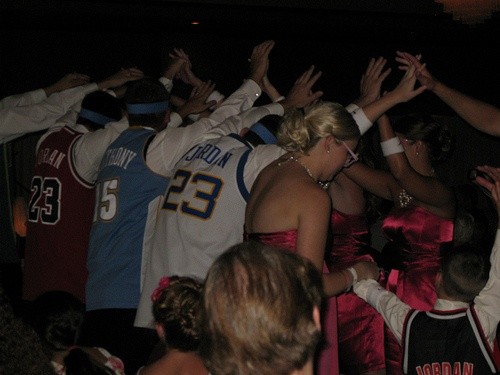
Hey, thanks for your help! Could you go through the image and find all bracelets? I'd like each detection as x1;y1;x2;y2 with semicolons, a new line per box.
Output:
342;271;350;291
348;267;357;284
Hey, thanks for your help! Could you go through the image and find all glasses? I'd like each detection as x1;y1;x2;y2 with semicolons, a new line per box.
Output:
336;138;359;167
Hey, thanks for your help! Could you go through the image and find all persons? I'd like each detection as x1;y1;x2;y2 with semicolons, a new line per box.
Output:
200;243;322;375
137;275;213;374
23;57;186;304
353;165;500;375
82;41;273;375
238;44;383;375
73;49;225;124
0;67;144;145
301;88;454;375
22;289;125;375
395;0;500;137
1;316;45;375
130;54;427;375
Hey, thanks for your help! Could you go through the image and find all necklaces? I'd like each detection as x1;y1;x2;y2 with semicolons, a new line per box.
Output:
276;155;330;191
397;166;434;207
